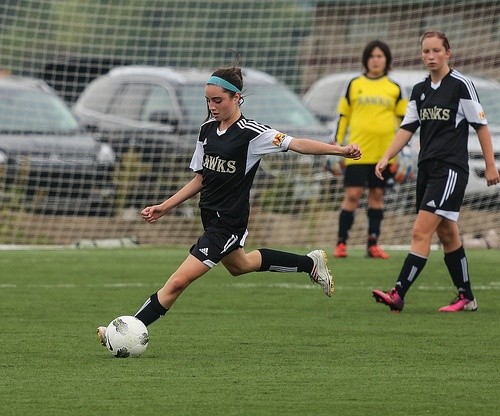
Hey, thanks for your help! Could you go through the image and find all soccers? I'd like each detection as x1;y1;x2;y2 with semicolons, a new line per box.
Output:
105;316;149;356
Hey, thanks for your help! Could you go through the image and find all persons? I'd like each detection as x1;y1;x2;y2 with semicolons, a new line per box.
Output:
96;68;362;346
372;32;500;313
325;40;409;260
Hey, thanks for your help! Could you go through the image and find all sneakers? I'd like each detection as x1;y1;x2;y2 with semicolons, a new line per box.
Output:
372;288;404;313
96;326;106;346
306;250;335;298
334;243;348;257
367;245;389;258
440;292;478;313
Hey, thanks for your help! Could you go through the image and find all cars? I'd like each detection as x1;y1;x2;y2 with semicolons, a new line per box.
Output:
70;63;334;214
300;69;500;216
0;76;122;217
